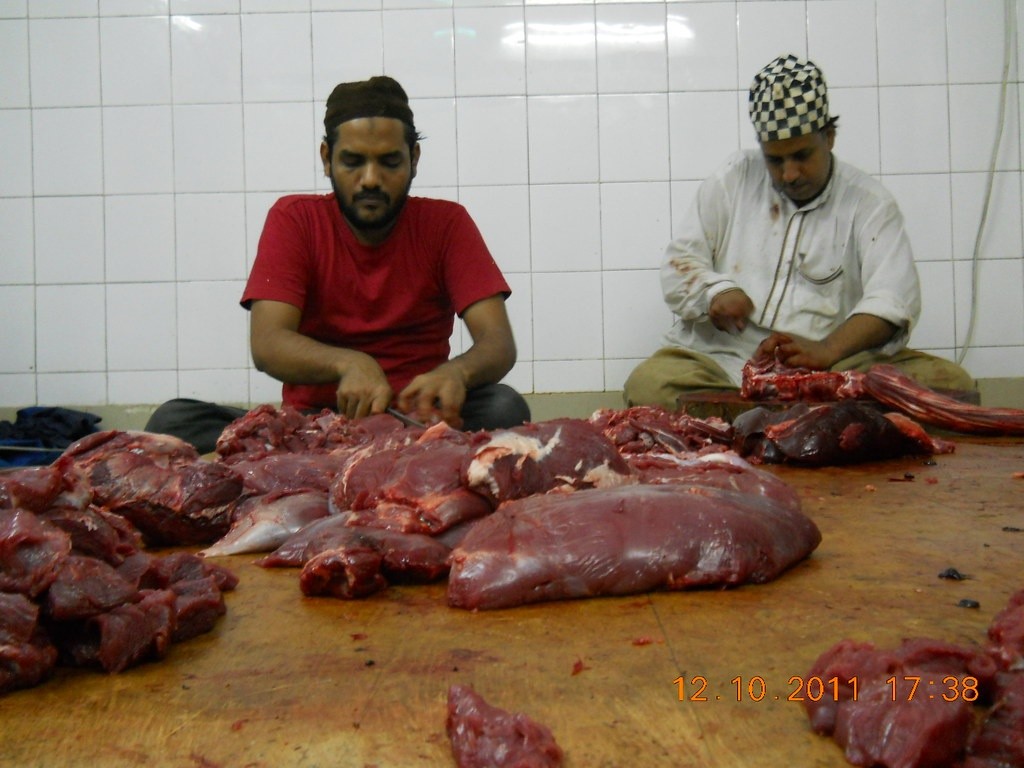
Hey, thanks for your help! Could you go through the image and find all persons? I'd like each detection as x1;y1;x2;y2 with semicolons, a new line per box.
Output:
142;76;532;456
623;54;982;419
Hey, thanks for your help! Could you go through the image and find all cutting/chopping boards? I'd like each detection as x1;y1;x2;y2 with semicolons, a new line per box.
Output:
678;388;984;426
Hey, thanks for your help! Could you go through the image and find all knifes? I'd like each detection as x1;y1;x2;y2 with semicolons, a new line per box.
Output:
386;406;426;429
744;316;795;346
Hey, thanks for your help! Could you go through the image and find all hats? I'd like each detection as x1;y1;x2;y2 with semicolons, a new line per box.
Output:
324;76;414;135
749;54;829;141
